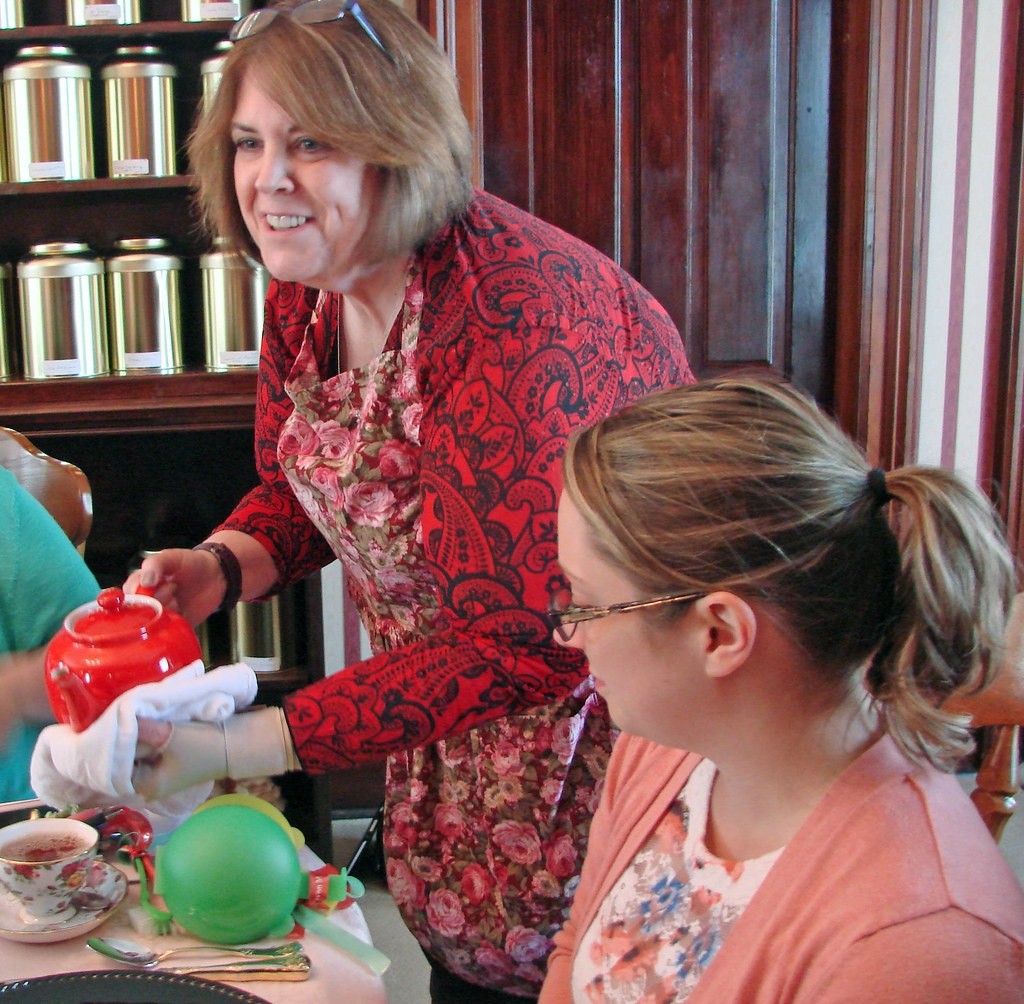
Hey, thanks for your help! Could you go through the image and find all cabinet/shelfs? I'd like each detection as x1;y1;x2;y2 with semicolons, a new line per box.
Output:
0;0;333;866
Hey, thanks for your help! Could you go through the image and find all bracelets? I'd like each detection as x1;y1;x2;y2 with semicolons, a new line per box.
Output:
194;539;244;611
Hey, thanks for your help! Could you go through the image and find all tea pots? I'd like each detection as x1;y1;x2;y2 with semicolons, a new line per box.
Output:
43;576;204;734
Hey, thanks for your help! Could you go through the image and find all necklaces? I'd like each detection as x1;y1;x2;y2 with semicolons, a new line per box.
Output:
329;289;351;375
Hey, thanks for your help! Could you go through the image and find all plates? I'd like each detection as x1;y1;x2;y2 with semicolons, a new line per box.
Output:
0;860;130;944
0;967;274;1004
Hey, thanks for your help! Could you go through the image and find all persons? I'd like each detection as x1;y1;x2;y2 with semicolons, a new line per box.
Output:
0;459;102;819
537;372;1024;1003
130;0;704;1004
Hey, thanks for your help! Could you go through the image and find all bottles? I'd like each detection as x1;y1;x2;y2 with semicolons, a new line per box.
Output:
0;0;270;382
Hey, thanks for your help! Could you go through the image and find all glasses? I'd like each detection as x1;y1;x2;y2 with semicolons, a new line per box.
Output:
546;586;774;642
229;0;393;62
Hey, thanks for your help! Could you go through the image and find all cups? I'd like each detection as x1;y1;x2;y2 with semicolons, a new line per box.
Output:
231;595;282;674
0;818;100;926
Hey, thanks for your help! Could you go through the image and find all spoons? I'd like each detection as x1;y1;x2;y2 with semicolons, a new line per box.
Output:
85;935;304;969
70;890;111;910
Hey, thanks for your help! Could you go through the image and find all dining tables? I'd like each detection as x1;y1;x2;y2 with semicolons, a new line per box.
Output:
0;843;390;1004
